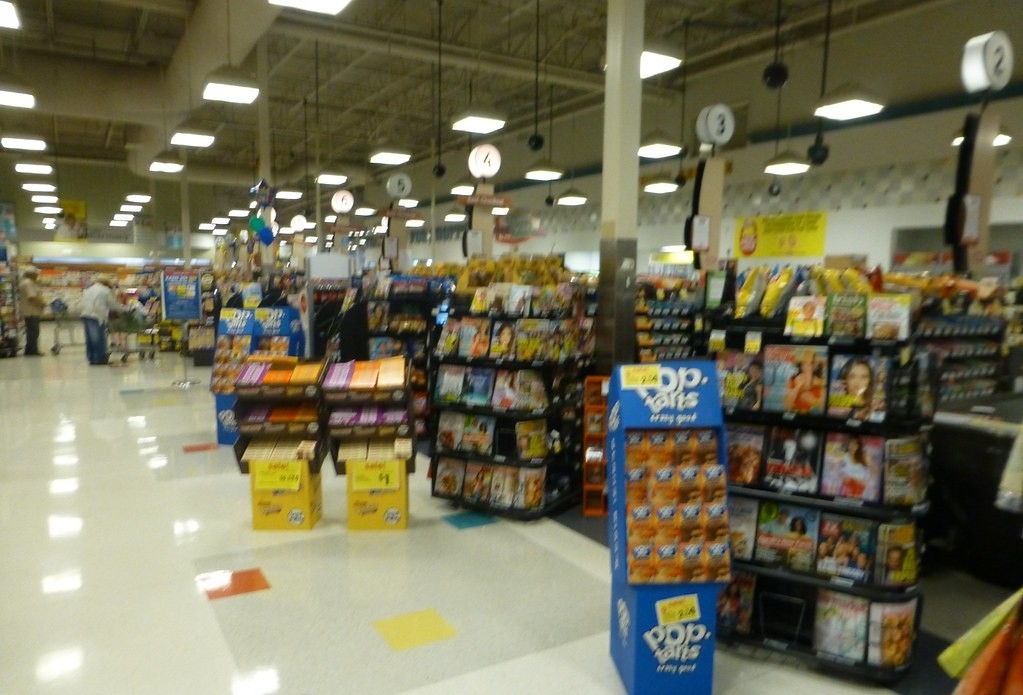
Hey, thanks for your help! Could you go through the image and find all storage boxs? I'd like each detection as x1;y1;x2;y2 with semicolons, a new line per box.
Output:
627;428;733;587
582;375;610;518
214;335;411;533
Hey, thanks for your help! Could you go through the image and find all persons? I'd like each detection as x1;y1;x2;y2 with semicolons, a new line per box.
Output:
503;372;516;400
369;302;387;329
738;360;763;412
492;322;514;357
470;319;490;355
759;509;790;536
784;360;824;410
833;357;874;414
551;332;560;360
474;470;484;490
81;276;121;364
885;547;905;573
786;516;810;563
832;437;875;498
19;265;47;355
789;301;823;337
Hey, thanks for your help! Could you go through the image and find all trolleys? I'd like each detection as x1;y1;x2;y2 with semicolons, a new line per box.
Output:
43;301;88;356
102;310;163;364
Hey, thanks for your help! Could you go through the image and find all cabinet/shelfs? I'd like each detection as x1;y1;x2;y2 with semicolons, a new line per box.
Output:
607;360;734;695
319;355;417;477
253;306;307;359
232;356;328;475
0;261;25;358
213;306;255;446
428;294;576;521
361;291;437;442
706;314;934;688
635;312;695;364
912;317;1007;436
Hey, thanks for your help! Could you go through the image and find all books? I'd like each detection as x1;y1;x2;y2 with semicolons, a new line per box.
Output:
432;283;594;509
366;301;392;360
784;291;909;340
720;346;926;665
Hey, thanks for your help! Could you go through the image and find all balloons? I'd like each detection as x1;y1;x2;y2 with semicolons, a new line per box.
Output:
249;215;265;232
258;228;273;245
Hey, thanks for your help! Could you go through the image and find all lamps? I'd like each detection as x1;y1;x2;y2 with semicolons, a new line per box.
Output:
124;129;152;203
0;81;40;109
812;0;886;121
147;36;186;174
523;84;565;182
449;104;508;136
597;36;685;79
443;198;468;223
169;15;216;148
200;0;260;105
555;166;590;208
367;146;413;166
193;169;427;253
643;159;679;195
0;0;25;31
0;129;49;153
636;74;685;159
448;175;475;197
761;0;810;177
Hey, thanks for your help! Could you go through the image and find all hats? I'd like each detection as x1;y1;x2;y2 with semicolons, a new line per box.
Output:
96;273;110;285
25;265;42;274
